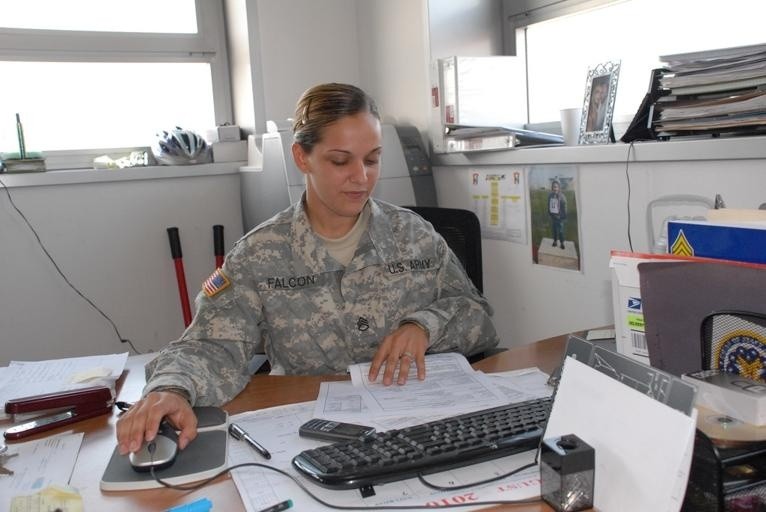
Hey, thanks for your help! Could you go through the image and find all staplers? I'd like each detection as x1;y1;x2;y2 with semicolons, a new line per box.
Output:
3;386;113;440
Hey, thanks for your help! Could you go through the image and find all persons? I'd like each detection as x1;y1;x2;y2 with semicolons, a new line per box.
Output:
547;177;569;251
596;77;609;132
114;80;501;457
585;81;604;132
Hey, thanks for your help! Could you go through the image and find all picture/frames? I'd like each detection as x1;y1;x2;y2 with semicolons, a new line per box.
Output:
577;58;621;144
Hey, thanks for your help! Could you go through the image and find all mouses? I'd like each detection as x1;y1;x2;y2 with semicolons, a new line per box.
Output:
126;422;179;471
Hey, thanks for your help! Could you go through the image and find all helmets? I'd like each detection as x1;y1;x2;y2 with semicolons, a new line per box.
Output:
151;127;211;165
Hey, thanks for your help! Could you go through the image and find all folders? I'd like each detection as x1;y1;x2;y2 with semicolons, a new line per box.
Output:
442;57;566;154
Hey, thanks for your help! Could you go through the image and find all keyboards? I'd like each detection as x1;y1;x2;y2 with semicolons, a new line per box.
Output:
291;395;554;498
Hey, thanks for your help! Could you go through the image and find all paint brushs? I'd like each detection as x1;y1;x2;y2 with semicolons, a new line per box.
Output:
253;499;294;510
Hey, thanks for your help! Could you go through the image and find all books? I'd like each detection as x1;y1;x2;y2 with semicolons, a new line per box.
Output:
650;42;766;140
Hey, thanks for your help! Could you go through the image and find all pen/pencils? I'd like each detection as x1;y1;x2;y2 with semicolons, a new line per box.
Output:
115;402;169;431
229;422;269;460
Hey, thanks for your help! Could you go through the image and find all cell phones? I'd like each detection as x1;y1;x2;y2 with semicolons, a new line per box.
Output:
298;417;377;443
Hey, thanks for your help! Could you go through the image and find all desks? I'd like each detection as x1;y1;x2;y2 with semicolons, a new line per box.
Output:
0;324;766;512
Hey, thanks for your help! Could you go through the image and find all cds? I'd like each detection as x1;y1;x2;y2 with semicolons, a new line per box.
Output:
704;412;746;431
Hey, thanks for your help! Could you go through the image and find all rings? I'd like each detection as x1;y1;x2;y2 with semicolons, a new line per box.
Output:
400;350;415;359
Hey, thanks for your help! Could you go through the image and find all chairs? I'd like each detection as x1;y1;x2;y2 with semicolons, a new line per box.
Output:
401;206;487;363
